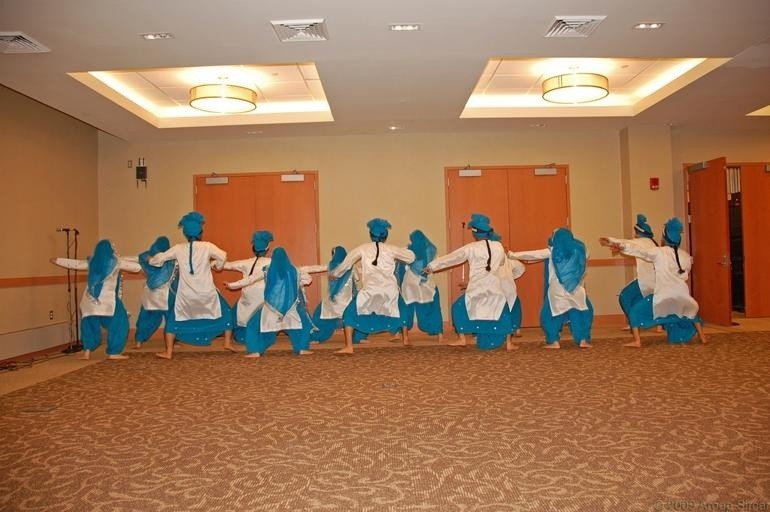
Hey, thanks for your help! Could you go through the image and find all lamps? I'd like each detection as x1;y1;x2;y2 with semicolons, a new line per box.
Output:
188;82;260;113
540;72;611;105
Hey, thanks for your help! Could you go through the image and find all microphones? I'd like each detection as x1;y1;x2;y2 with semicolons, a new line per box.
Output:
73;228;79;234
56;228;71;232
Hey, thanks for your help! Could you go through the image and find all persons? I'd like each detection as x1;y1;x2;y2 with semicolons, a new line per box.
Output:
118;235;175;349
388;230;444;344
210;231;273;345
610;218;706;347
298;246;372;344
49;238;142;360
145;212;240;360
599;213;665;333
331;217;415;356
420;214;594;351
222;247;315;358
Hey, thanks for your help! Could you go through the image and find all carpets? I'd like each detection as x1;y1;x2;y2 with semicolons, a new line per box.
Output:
0;327;770;512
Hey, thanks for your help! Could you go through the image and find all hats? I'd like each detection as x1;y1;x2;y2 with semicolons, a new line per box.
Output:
662;224;682;246
368;225;389;242
633;220;655;238
182;218;203;242
473;221;495;238
253;238;270;256
